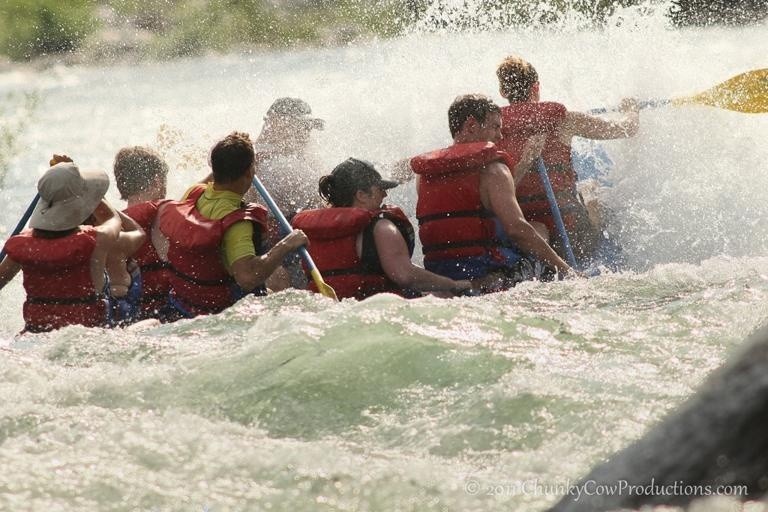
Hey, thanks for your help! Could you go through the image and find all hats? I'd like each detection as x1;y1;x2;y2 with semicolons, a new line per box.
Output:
331;157;398;197
29;162;109;231
262;97;327;131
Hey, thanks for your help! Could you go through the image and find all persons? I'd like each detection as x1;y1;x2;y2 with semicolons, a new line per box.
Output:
82;196;146;328
161;130;310;323
495;56;640;273
292;156;473;303
0;153;121;333
113;146;175;325
242;97;333;293
411;93;583;297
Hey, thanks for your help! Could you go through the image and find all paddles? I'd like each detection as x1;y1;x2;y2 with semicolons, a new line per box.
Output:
253;172;341;302
583;68;767;112
